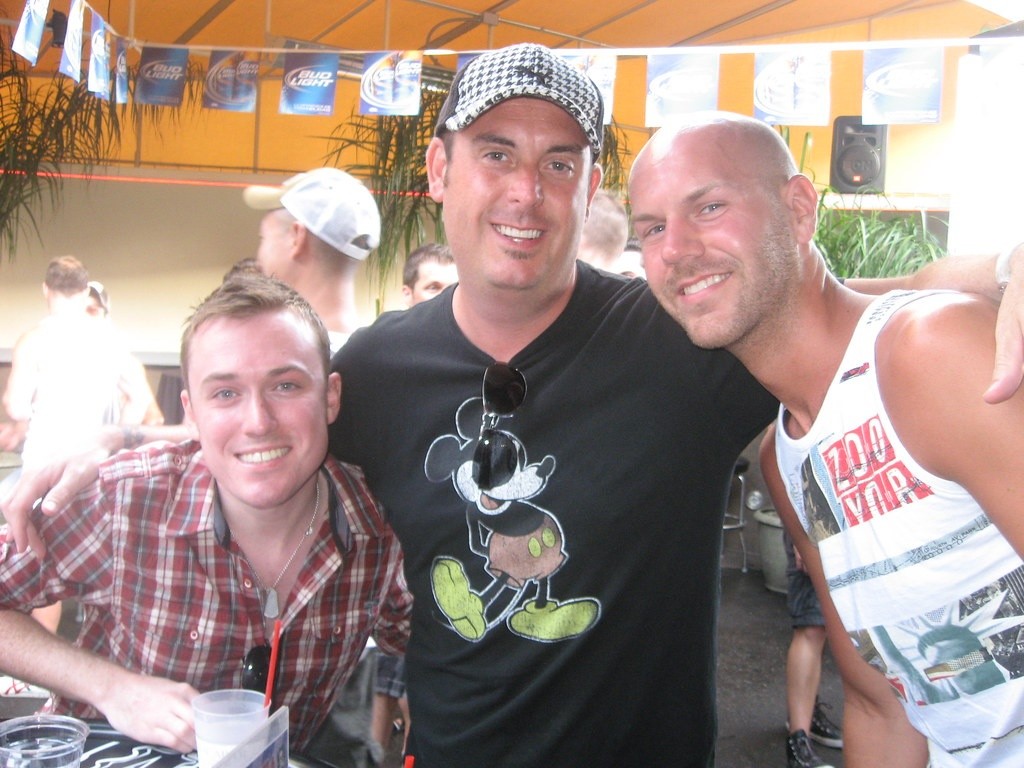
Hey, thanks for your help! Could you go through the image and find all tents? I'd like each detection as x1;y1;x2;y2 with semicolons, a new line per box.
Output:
0;0;1012;202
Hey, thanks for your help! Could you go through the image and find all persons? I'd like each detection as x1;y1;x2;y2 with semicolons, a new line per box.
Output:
784;528;844;768
0;40;1024;768
243;166;381;360
3;255;165;477
627;110;1024;768
401;242;459;307
0;276;413;754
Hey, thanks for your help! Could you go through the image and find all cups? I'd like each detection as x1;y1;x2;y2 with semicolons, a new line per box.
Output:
191;689;271;768
0;715;89;768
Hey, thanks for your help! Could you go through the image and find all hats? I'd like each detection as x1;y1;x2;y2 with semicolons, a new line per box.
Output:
433;41;606;171
88;280;110;314
242;166;383;260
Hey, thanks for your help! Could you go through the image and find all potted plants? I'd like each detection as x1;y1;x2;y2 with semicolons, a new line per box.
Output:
752;122;952;595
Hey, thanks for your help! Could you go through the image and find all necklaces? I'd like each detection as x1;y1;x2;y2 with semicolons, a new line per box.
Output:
217;478;319;619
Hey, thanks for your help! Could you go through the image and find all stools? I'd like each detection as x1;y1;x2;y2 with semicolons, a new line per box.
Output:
723;454;752;573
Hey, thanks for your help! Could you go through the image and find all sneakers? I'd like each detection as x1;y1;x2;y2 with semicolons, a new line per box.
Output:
810;695;844;748
785;728;835;768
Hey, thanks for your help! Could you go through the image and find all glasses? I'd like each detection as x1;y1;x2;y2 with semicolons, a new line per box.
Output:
471;361;529;491
241;640;281;714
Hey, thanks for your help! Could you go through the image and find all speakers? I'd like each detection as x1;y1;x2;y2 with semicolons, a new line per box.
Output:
830;116;887;195
